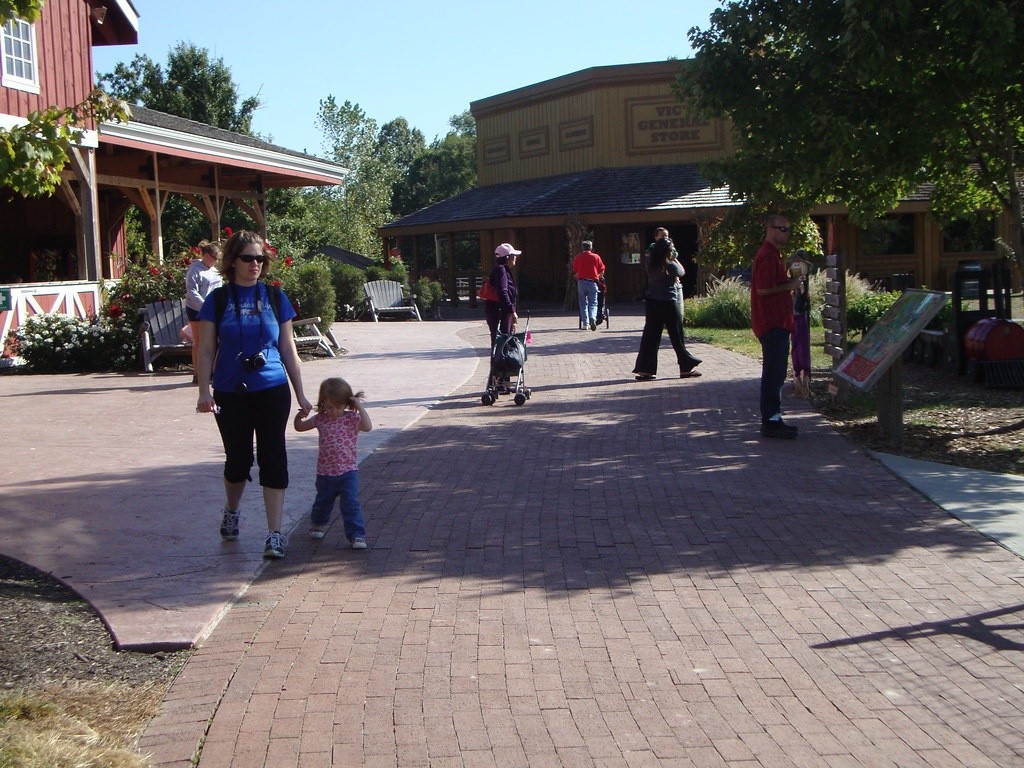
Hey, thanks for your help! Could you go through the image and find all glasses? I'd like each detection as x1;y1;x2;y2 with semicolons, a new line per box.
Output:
236;253;266;263
771;224;792;233
208;253;221;263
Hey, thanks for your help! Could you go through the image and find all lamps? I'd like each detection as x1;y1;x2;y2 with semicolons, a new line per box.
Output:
90;5;107;24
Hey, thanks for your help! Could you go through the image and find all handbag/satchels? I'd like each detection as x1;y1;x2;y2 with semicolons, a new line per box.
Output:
493;335;526;372
479;282;501;302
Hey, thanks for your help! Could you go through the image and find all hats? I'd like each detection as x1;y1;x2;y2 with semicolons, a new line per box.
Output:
495;243;522;258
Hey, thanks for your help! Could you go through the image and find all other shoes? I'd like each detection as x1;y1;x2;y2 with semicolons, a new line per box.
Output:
690;355;703;367
192;376;198;384
582;325;588;329
209;374;214;383
589;318;596;331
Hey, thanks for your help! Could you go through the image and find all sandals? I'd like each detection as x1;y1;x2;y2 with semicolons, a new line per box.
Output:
635;371;656;380
680;371;702;378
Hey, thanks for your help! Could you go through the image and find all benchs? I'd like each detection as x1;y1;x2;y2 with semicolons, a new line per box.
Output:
137;299;193;372
359;280;423;323
288;316;336;358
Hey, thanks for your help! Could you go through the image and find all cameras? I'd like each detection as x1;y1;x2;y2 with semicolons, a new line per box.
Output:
243;352;267;372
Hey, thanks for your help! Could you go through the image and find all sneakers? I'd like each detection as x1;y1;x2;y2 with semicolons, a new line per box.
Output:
760;419;798;438
264;530;288;559
219;504;245;540
352;536;367;549
308;520;324;538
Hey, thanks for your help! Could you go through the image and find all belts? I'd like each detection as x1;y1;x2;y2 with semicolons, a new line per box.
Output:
578;278;594;282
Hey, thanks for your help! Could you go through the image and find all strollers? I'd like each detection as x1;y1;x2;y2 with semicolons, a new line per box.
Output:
481;309;532;406
577;276;610;330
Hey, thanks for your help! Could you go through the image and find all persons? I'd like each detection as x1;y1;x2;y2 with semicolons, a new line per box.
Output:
185;244;222;385
786;249;818;399
632;227;703;381
484;243;521;395
196;230;312;558
295;378;372;548
572;240;605;331
751;215;802;438
